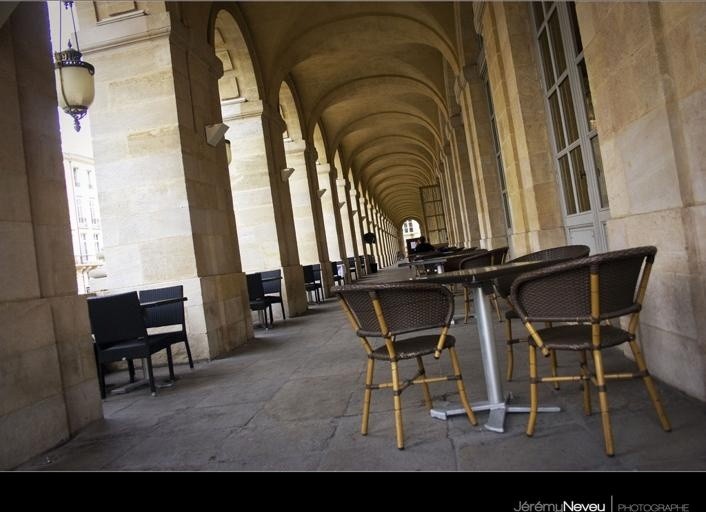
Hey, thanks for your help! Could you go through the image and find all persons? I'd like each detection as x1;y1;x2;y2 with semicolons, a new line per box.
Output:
416;236;433;252
414;238;421;252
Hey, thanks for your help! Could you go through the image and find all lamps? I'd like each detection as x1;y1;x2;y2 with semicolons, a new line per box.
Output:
224;138;231;166
52;1;95;133
